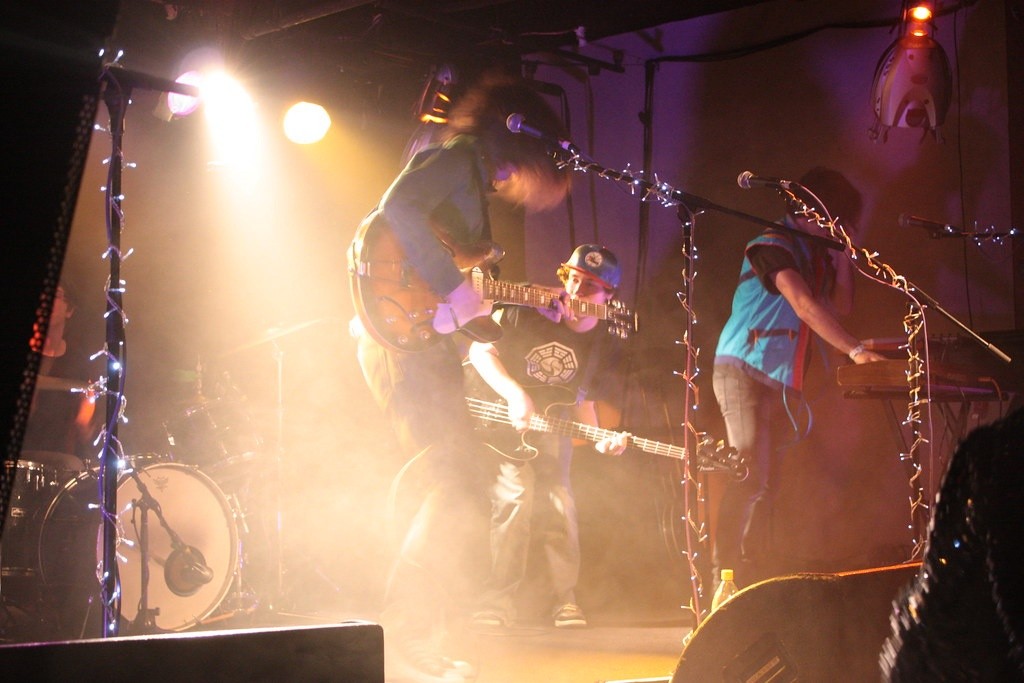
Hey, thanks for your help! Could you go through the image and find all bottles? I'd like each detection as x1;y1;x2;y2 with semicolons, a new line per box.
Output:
711;568;740;615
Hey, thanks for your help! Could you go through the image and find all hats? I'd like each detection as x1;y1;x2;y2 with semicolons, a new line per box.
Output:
561;244;618;289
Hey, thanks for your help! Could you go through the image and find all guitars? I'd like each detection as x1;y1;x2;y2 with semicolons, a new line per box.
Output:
348;208;638;354
458;359;749;484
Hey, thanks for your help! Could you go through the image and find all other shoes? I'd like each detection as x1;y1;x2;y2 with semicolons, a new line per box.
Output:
551;602;587;627
474;607;508;625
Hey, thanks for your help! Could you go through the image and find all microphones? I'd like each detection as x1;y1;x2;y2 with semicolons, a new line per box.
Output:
737;170;799;190
190;567;214;586
898;213;955;233
505;113;579;154
100;66;198;96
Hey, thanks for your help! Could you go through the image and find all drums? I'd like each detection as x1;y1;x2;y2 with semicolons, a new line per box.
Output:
1;460;43;578
37;454;238;635
162;395;255;476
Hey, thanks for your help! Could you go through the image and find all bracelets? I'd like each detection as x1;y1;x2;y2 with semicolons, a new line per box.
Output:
849;344;866;359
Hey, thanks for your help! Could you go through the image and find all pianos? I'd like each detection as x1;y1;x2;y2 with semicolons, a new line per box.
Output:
834;361;1007;562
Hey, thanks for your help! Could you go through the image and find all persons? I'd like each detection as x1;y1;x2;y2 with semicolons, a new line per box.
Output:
468;244;628;627
19;269;96;482
712;167;889;599
356;77;572;683
879;403;1024;683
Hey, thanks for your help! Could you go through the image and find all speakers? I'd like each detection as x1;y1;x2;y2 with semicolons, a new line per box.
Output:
671;562;924;683
569;425;709;626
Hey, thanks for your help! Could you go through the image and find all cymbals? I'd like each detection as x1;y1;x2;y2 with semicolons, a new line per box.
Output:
224;317;322;358
33;371;90;393
